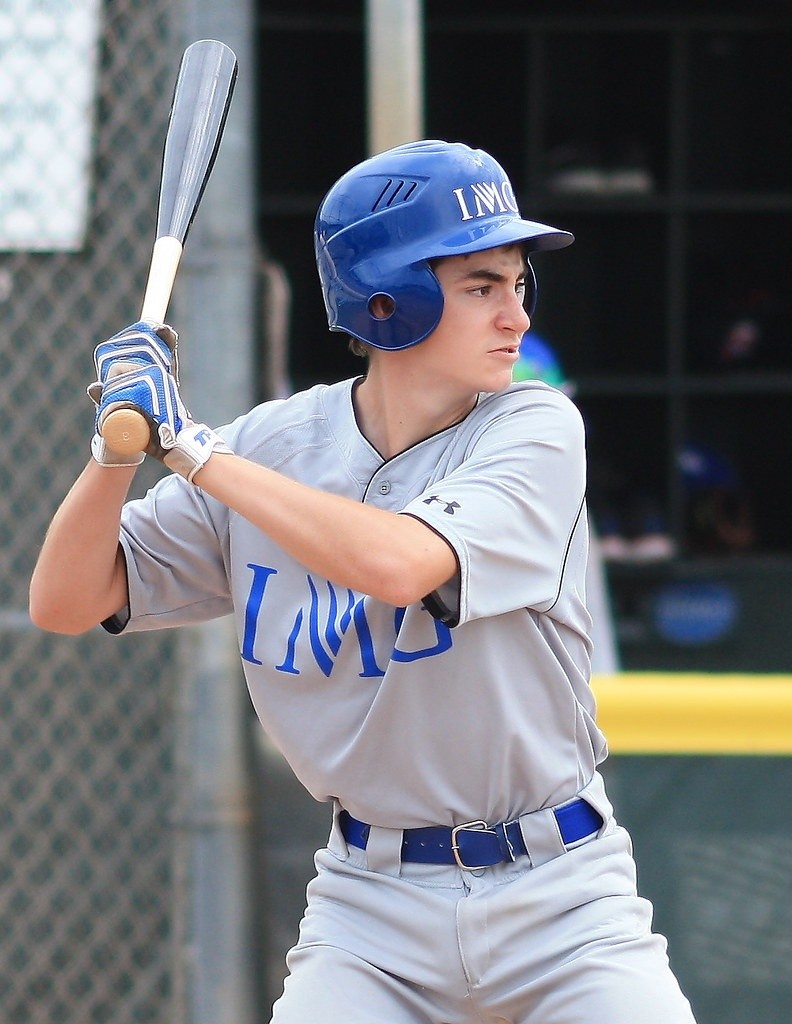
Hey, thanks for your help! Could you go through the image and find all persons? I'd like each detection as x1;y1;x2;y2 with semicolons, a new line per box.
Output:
28;138;694;1024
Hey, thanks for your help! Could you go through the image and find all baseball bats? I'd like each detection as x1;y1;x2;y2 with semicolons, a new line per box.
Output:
102;40;237;454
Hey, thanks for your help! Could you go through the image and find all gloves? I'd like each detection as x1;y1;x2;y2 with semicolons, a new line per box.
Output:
90;319;178;466
86;367;236;488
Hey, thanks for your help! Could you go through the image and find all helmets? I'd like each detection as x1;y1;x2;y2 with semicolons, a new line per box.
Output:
312;138;575;352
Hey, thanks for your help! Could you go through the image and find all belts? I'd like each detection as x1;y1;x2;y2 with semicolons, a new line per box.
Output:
337;798;604;871
